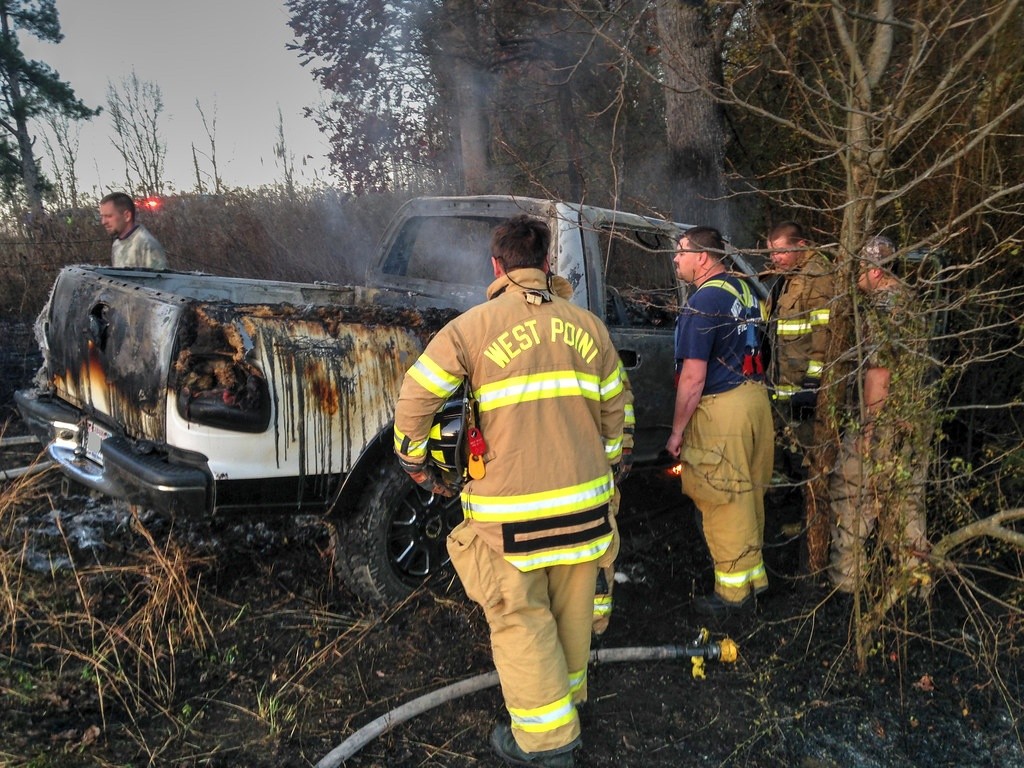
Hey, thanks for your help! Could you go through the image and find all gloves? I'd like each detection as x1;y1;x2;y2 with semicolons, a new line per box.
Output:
791;379;820;408
613;448;633;485
399;458;453;498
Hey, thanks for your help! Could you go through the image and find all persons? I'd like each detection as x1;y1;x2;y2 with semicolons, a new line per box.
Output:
666;227;774;615
766;221;932;612
100;193;170;270
392;214;635;768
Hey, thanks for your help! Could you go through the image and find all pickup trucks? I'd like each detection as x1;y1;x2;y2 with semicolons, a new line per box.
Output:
3;193;773;614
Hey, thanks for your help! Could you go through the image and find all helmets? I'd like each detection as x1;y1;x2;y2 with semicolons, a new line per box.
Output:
426;377;475;483
859;235;896;265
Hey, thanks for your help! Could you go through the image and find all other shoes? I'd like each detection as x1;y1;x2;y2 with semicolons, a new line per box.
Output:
693;592;755;617
492;724;582;768
820;571;854;599
752;585;769;599
780;520;803;541
589;631;603;650
